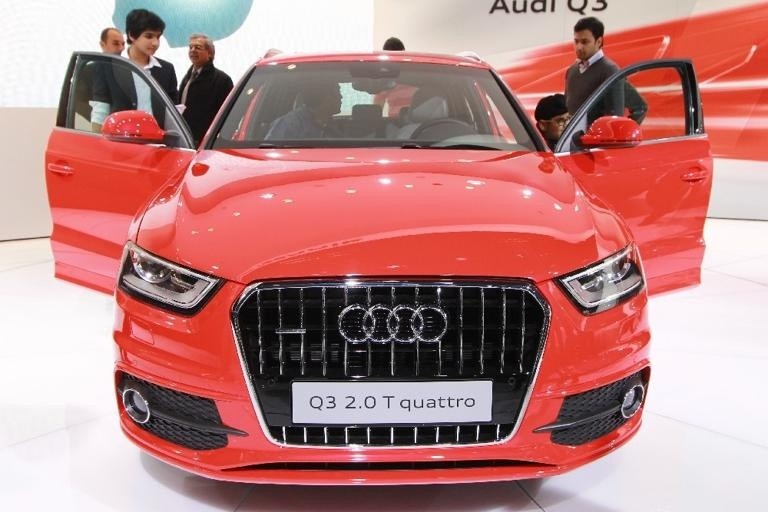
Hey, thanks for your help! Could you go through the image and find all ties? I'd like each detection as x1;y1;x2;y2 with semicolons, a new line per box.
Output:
579;60;589;73
181;70;198;106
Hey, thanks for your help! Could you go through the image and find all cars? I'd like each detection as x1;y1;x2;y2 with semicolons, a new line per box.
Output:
44;47;713;486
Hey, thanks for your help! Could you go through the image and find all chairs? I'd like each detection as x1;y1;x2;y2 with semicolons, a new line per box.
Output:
394;87;453;142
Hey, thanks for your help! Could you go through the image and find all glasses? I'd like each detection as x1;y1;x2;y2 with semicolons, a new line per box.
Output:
541;118;571;127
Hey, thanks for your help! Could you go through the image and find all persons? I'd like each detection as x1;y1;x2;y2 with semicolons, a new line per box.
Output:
87;8;182;132
371;37;422;120
264;76;345;142
176;30;235;145
74;28;126;120
625;80;649;125
532;91;574;156
561;16;626;133
